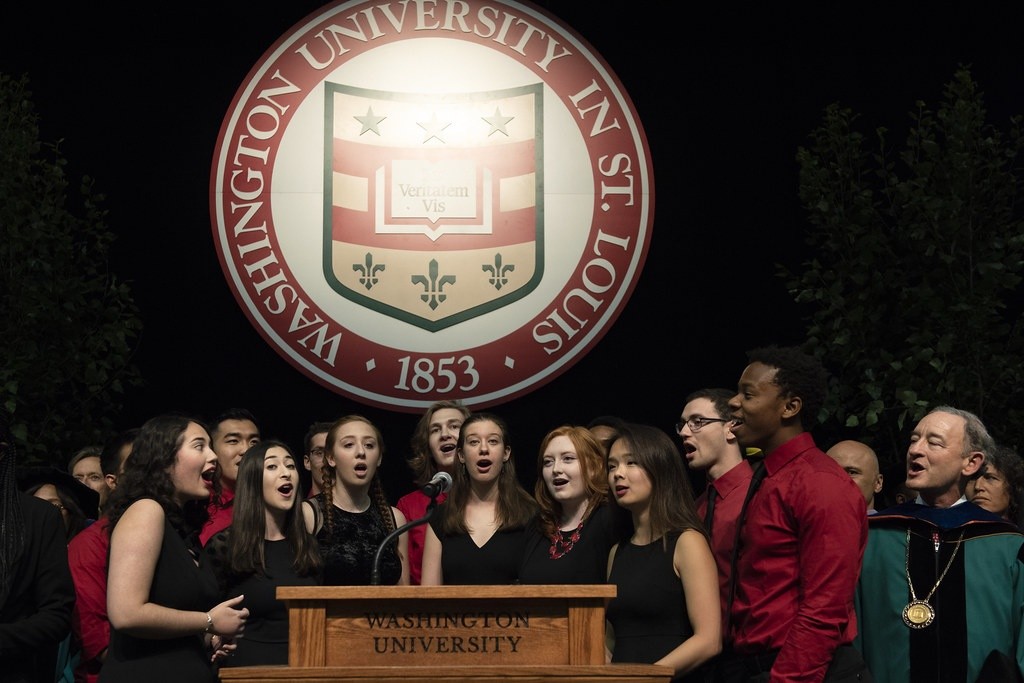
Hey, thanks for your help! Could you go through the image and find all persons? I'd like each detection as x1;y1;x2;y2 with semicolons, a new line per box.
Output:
0;345;1024;683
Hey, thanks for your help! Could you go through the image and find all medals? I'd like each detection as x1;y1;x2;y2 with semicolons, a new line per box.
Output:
902;600;934;630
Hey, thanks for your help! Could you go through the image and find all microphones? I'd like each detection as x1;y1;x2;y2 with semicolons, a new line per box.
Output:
423;471;453;497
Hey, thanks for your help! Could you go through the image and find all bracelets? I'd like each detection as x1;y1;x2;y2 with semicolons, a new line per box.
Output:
205;612;214;633
210;634;215;645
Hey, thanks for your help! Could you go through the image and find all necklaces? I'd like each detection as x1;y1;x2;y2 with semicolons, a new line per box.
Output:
548;519;584;560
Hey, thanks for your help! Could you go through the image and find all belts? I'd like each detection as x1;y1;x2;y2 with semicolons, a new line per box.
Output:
727;647;783;679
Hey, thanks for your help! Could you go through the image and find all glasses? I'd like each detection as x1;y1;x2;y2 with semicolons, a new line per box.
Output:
675;416;727;435
307;448;326;460
